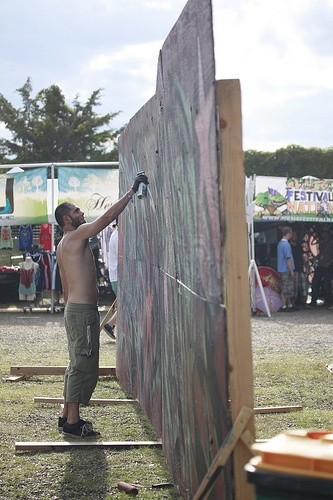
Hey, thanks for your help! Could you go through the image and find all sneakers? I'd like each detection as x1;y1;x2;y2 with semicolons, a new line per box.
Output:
103;323;117;339
58;415;102;438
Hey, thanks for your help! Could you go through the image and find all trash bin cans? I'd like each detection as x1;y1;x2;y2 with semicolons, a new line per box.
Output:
245;426;333;500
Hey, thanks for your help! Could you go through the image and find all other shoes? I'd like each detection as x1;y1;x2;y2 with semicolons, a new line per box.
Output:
280;304;297;313
305;303;317;310
